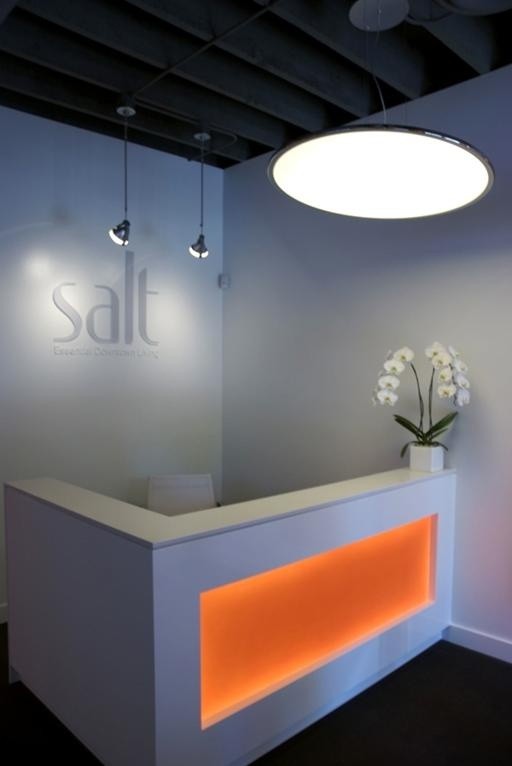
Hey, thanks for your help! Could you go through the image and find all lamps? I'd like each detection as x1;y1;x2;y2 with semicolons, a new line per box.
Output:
108;102;136;249
189;131;211;260
265;0;496;222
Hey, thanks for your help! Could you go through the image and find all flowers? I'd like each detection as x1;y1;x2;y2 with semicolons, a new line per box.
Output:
369;340;474;459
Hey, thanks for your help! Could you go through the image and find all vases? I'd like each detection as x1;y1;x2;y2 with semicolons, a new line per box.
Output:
407;444;446;476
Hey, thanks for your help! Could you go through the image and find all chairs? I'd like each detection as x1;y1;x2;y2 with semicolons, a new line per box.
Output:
146;474;223;517
